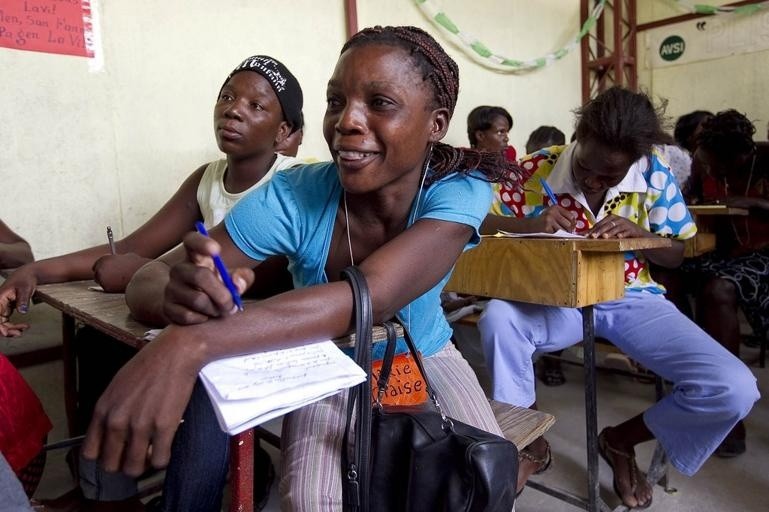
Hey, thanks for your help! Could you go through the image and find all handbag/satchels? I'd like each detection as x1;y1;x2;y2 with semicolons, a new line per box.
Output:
342;265;519;512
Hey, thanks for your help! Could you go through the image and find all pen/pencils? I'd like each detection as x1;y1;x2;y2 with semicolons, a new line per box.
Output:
106;226;118;257
196;220;244;310
539;177;559;205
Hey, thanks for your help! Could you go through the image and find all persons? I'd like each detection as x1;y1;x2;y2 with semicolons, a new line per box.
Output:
468;84;769;511
0;54;306;510
0;217;54;502
82;22;507;512
272;110;304;157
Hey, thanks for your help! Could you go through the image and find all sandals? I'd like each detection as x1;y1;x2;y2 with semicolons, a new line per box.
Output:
597;426;652;510
515;435;554;498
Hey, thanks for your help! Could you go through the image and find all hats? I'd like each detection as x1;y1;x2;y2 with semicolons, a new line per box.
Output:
217;55;303;127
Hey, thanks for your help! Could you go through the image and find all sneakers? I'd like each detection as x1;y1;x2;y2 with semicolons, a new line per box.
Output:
714;419;746;457
534;356;567;386
